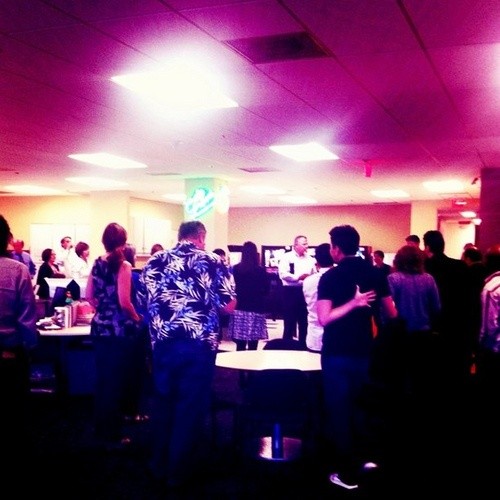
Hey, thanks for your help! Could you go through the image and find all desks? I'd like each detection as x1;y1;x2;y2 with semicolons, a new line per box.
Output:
39;326;93;395
44;276;88;299
215;350;323;462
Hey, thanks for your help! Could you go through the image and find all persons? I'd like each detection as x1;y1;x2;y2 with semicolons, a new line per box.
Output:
227;242;276;351
150;244;163;256
355;231;500;456
137;222;237;479
303;244;377;353
124;244;136;268
317;226;398;489
85;223;140;451
0;214;42;500
11;240;36;280
71;243;92;299
57;237;75;288
36;249;60;300
213;249;225;257
278;236;317;343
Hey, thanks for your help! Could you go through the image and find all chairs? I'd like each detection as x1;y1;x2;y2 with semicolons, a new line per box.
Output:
66;279;80;301
44;286;68;318
263;338;311;351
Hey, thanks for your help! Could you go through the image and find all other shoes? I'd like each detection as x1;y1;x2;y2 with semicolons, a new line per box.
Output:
364;461;378;468
330;474;358;489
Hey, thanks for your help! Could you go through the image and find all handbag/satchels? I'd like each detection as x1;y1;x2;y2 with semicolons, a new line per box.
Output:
219;298;237;314
